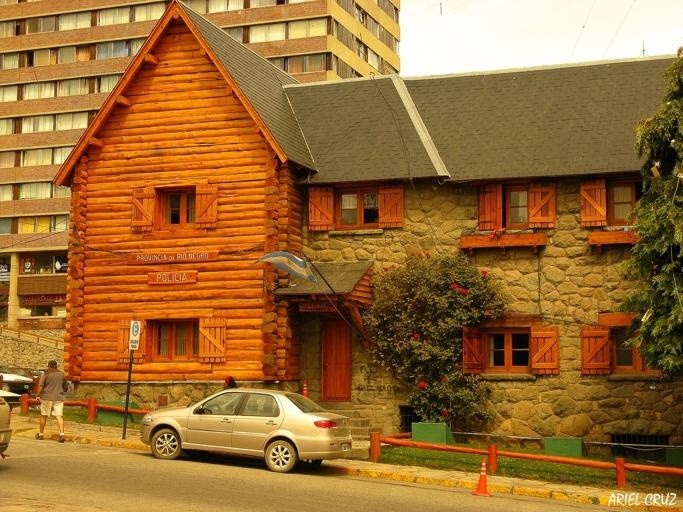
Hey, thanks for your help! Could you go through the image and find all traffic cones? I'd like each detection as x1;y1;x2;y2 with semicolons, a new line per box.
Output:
470;458;491;498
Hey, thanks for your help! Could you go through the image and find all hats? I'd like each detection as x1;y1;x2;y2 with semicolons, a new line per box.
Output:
47;360;57;368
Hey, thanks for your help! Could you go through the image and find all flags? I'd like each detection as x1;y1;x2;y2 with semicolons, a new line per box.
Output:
252;250;317;284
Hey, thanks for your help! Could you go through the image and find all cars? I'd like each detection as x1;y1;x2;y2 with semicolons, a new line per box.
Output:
139;386;353;473
0;395;12;460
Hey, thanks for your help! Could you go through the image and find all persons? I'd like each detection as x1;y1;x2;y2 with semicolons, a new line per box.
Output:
35;359;68;443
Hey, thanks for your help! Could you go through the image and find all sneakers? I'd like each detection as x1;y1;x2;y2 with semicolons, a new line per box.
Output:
59;435;65;442
35;433;43;440
